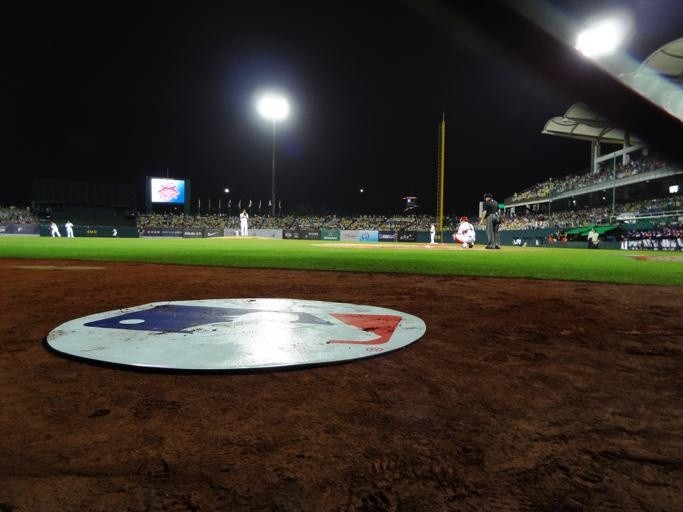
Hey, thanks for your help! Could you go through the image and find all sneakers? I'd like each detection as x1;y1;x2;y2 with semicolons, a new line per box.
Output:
486;246;492;248
462;243;468;247
495;245;500;248
469;243;474;248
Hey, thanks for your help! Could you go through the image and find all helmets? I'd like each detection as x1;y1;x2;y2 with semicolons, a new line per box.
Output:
460;217;468;221
485;193;492;198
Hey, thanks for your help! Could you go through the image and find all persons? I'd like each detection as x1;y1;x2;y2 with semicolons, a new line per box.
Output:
63;221;75;237
84;226;98;237
134;211;475;248
111;226;118;237
0;206;57;226
477;157;682;252
47;220;61;237
238;208;249;236
